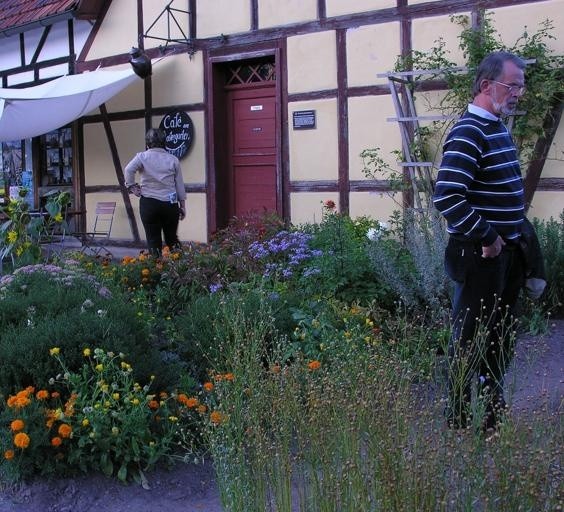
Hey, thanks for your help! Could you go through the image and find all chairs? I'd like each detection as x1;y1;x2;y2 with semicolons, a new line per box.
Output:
72;202;116;258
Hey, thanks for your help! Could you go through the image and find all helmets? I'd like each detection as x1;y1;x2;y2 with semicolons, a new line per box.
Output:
145;128;166;146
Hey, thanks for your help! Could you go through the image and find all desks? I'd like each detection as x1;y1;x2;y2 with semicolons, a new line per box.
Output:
28;210;78;266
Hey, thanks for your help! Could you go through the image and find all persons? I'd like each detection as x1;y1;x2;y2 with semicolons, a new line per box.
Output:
433;50;527;430
125;128;187;256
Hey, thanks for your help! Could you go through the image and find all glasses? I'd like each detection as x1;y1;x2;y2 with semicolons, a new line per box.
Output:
487;78;528;98
